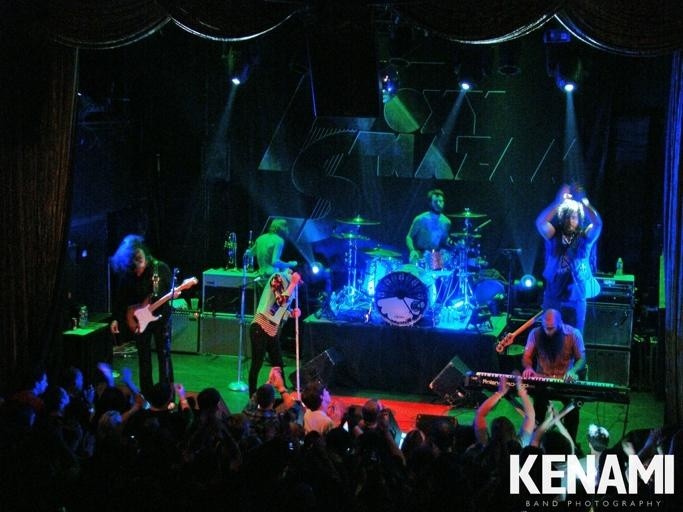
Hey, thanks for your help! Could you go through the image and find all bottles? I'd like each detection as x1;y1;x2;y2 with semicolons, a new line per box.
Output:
78;305;89;328
616;257;624;277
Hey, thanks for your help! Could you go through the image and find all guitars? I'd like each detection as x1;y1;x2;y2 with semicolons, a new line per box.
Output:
497;309;545;353
127;277;198;337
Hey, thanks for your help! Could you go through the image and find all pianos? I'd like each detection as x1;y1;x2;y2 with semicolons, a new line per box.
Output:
476;369;632;404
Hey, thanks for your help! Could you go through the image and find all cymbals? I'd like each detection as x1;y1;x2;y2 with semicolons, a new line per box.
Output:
447;214;487;218
366;250;402;255
341;219;381;227
336;232;367;240
448;233;482;239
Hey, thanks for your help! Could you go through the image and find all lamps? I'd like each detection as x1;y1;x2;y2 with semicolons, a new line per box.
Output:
229;57;251;84
552;58;583;95
459;68;486;91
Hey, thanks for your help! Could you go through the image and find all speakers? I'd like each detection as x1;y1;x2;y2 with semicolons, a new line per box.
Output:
62;323;113;385
583;348;631;387
428;352;475;403
416;414;457;437
348;405;402;450
585;302;635;349
288;347;336;389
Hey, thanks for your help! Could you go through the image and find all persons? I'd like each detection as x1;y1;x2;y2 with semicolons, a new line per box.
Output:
245;217;299;280
406;187;456;260
521;309;588;441
107;240;177;394
249;264;304;398
1;364;683;511
534;178;604;369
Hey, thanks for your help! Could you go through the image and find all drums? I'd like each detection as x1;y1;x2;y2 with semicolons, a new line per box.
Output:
376;266;435;326
368;256;402;293
421;250;457;273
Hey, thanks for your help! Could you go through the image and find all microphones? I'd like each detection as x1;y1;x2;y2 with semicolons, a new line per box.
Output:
287;268;304;285
252;276;262;281
152;258;160;296
